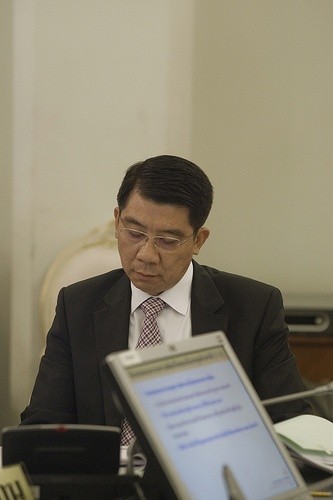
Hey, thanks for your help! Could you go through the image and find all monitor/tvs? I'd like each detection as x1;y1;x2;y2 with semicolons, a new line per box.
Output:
102;330;308;500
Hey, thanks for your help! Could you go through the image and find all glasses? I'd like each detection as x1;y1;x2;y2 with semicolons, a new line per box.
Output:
117;210;197;254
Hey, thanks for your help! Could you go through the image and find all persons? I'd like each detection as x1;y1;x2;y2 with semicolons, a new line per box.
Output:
19;156;311;500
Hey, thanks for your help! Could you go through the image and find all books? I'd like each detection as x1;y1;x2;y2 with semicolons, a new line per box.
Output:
273;414;333;473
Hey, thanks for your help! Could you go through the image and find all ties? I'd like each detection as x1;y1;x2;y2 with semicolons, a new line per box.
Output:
120;298;169;446
